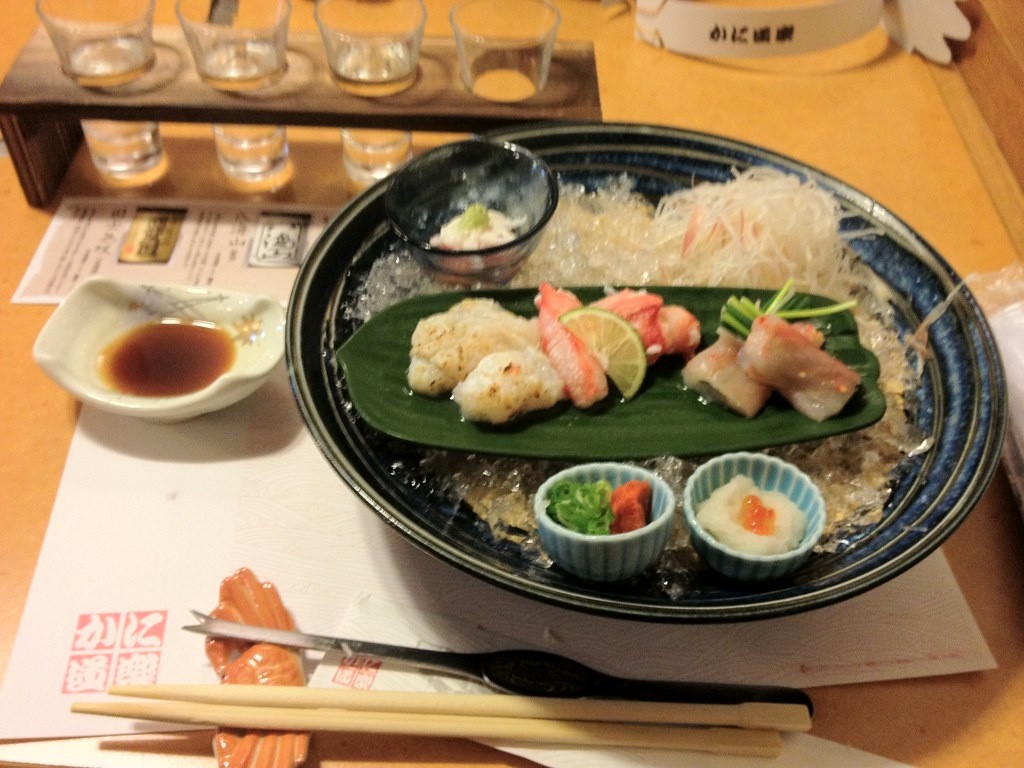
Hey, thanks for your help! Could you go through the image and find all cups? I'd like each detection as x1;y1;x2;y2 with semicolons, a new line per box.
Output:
450;2;561;143
172;1;290;180
37;0;166;182
314;1;425;180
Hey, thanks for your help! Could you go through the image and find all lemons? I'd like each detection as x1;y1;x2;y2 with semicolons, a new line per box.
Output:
559;307;645;401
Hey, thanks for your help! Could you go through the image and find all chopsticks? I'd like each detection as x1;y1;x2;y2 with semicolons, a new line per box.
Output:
68;683;814;758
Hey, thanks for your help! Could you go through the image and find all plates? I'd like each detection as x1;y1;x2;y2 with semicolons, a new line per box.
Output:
36;276;293;423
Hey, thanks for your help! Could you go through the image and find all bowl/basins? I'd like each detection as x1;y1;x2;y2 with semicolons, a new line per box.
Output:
283;115;1008;629
682;445;829;581
389;134;561;289
535;459;681;579
